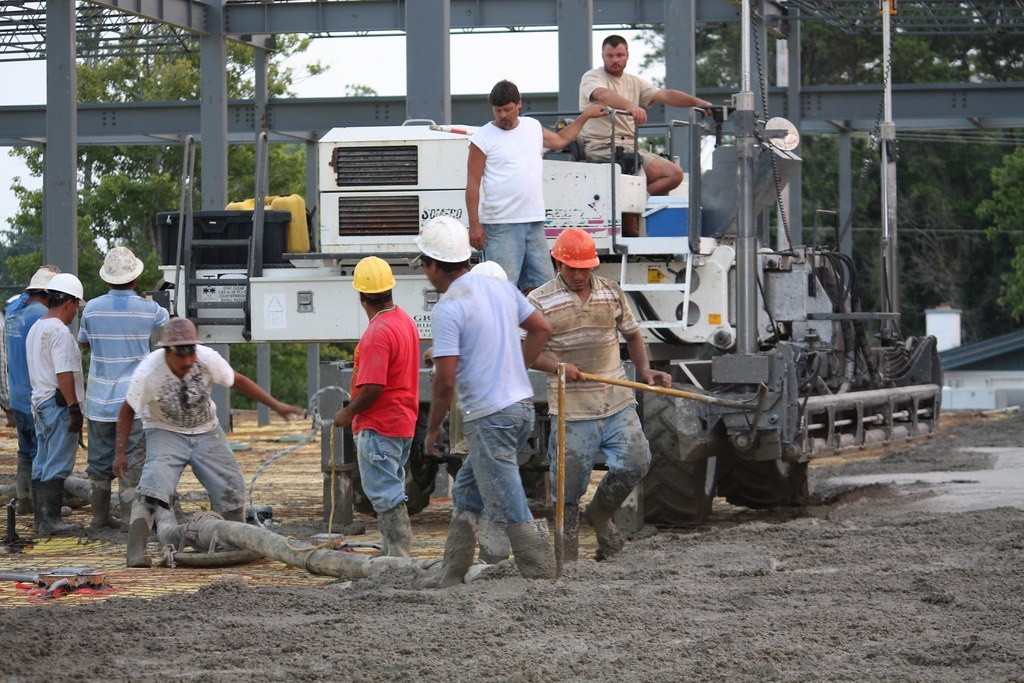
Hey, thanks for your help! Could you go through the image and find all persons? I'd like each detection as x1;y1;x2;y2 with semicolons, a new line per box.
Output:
333;255;421;560
3;265;72;517
25;273;83;536
465;80;609;296
77;247;170;536
416;215;557;591
112;318;306;568
519;228;672;564
578;35;716;238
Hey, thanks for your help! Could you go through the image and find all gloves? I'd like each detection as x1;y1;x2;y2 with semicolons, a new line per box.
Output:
66;404;83;433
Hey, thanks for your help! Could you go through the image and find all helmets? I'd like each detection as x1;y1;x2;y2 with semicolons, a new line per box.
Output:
352;256;396;294
25;268;58;291
413;216;472;263
151;317;202;348
99;246;144;285
470;261;509;282
550;228;600;268
44;273;87;308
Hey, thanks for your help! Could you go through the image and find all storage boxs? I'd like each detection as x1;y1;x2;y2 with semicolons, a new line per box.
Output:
157;209;290;269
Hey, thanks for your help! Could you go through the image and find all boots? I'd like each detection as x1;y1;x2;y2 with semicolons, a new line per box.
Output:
59;505;72;517
554;502;579;565
87;473;129;544
371;500;413;558
119;469;142;531
585;471;631;558
126;496;156;568
413;509;479;592
503;519;558;581
14;451;33;515
222;505;246;528
31;478;85;536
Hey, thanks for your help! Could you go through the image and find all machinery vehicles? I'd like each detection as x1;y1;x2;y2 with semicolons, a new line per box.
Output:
142;0;942;528
